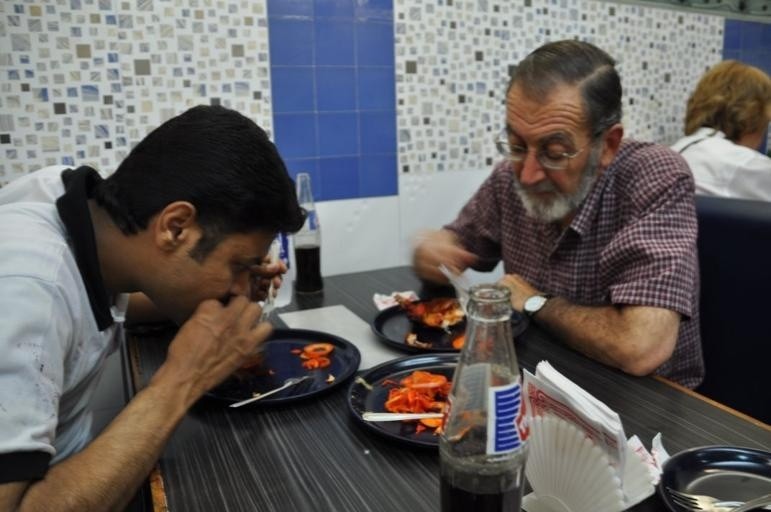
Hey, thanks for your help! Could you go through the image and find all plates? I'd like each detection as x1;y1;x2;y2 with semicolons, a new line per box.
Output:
207;328;361;412
346;354;460;446
658;446;771;512
370;297;528;356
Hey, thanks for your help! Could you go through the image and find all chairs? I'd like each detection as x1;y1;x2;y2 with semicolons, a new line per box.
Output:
694;195;770;425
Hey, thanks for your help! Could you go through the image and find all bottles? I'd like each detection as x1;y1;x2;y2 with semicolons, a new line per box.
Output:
439;285;531;510
293;171;321;293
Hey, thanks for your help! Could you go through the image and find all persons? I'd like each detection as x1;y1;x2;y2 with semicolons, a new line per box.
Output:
412;40;707;391
0;104;307;512
669;58;771;202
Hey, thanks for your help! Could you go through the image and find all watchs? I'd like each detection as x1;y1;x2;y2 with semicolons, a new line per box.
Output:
523;294;554;319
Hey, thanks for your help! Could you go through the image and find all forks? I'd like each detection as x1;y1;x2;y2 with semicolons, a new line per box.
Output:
667;487;771;512
259;235;281;328
227;367;334;410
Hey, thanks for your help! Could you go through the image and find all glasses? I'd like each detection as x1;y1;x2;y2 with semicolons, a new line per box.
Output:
494;124;597;170
